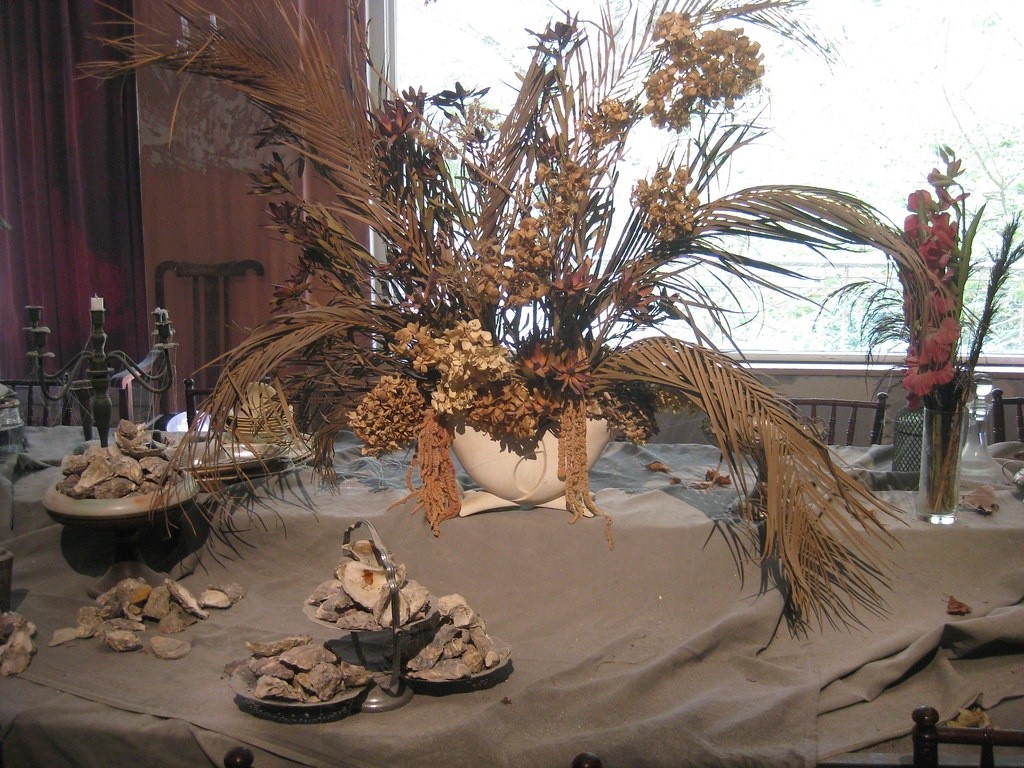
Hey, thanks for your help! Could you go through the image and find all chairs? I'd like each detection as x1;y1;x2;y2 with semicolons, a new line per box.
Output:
776;391;889;447
110;257;265;430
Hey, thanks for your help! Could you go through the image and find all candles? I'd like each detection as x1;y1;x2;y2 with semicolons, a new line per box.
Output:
91;293;104;310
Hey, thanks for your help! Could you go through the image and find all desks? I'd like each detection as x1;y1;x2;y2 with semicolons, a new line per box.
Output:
0;422;1024;768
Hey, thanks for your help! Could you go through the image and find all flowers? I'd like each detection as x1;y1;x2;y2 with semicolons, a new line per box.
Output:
813;146;1024;503
72;0;991;641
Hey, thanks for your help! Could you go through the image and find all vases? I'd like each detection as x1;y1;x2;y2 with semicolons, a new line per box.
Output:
915;406;964;523
452;415;610;521
